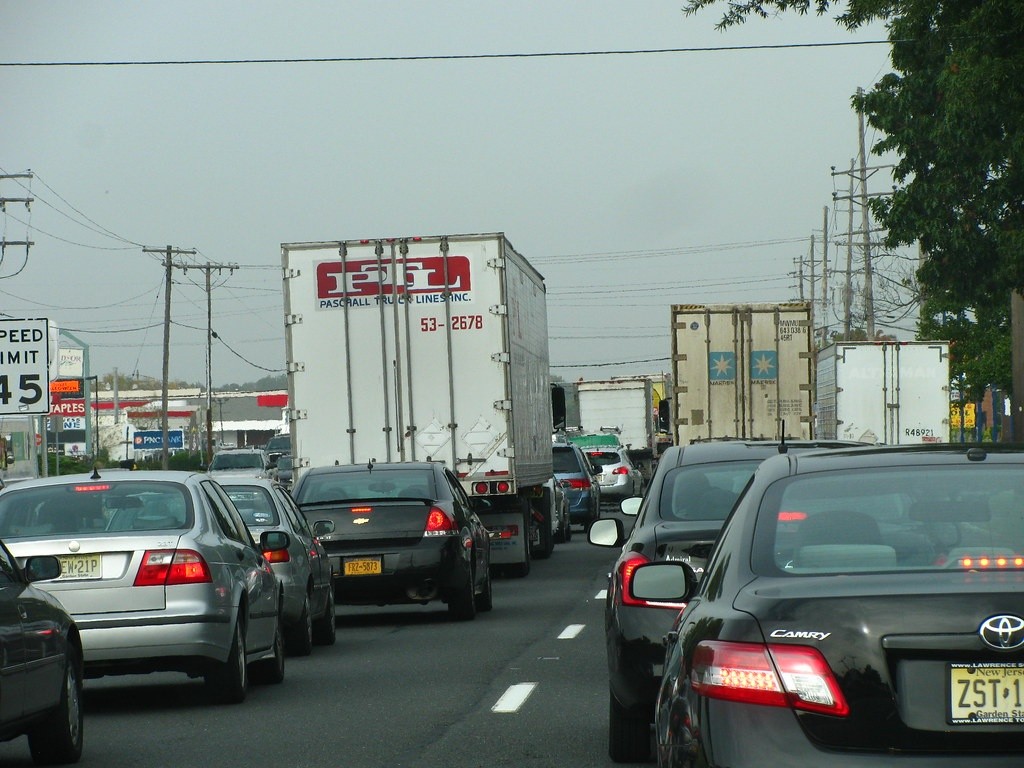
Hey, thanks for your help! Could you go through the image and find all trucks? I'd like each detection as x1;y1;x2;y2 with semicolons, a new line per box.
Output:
669;303;819;450
279;232;566;578
579;374;676;489
814;339;950;450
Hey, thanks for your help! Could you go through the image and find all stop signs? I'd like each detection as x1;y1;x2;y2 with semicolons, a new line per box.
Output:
35;434;42;445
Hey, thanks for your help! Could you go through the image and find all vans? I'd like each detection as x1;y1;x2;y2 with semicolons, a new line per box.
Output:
567;436;621;447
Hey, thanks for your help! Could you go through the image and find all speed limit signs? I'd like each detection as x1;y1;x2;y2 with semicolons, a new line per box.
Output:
0;318;54;418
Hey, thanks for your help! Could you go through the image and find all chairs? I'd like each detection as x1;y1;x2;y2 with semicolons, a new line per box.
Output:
794;543;896;568
8;522;55;537
793;510;883;546
398;488;431;499
942;548;1019;566
315;489;348;502
132;516;178;530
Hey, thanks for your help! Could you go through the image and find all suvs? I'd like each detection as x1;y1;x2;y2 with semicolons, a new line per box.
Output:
580;444;646;508
208;446;270;479
552;444;600;534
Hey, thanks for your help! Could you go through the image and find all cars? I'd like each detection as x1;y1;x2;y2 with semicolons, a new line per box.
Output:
265;433;295;488
0;540;84;765
652;440;1024;768
587;420;879;765
213;477;337;657
0;467;290;705
291;460;491;617
